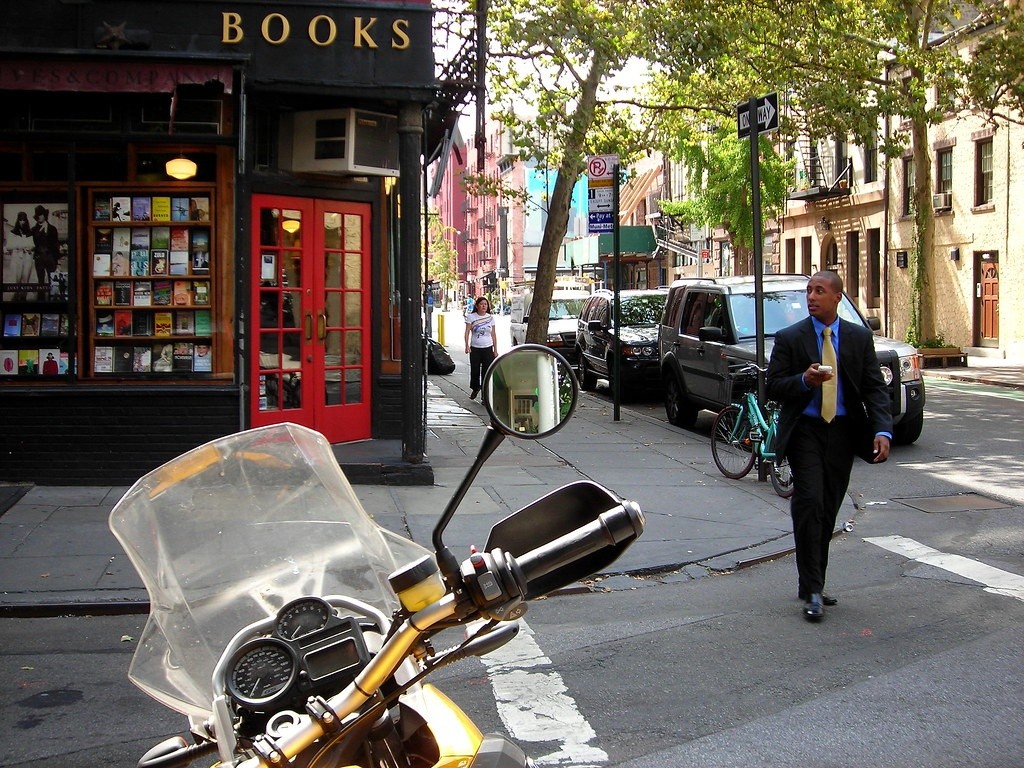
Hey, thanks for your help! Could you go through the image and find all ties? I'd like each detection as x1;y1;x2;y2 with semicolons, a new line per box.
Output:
820;327;837;424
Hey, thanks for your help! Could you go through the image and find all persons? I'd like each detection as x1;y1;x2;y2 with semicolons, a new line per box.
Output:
194;345;211;371
766;270;892;626
467;293;474;314
462;296;467;317
464;297;498;404
7;205;68;301
112;252;128;276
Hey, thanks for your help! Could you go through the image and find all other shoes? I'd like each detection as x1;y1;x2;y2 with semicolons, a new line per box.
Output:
471;386;481;399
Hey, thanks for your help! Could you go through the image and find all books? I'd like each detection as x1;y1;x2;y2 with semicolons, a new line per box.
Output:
0;310;78;375
94;197;212;372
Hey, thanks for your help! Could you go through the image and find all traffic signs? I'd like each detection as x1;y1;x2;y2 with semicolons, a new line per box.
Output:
737;91;780;140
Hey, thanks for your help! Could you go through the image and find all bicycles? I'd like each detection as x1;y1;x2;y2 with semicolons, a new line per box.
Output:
710;360;794;499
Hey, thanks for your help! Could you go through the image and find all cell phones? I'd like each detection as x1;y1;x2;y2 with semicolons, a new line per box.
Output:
818;366;832;374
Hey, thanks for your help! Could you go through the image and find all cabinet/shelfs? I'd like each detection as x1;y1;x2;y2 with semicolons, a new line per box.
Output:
87;187;216;378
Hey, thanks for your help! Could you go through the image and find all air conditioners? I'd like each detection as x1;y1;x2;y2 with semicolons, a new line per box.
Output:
931;194;952;210
291;107;401;177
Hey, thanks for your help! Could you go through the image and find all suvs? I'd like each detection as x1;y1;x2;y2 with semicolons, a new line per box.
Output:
657;273;926;454
574;286;671;403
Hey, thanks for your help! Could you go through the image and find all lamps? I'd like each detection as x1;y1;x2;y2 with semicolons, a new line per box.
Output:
165;153;198;180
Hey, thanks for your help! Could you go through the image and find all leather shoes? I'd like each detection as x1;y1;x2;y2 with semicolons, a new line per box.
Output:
804;592;824;620
801;594;837;605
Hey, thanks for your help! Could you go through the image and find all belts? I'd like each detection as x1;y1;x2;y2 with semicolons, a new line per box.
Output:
799;413;848;424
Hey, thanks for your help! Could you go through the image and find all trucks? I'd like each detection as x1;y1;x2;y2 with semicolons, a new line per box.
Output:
509;276;594;380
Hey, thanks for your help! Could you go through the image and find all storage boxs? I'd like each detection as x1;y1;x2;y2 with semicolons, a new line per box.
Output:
92;228;210;276
94;342;213;372
93;196;210;221
96;310;212;337
94;280;211;306
